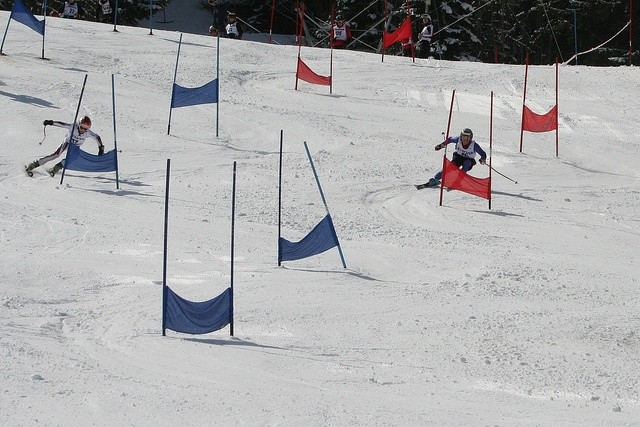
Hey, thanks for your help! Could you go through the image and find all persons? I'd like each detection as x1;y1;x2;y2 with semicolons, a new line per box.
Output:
331;14;353;48
224;13;242;40
401;37;415;56
418;13;434;58
95;0;115;25
63;0;79;20
208;25;218;37
26;116;105;177
428;128;487;191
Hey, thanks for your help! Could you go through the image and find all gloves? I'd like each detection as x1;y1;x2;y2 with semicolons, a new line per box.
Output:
98;145;104;155
43;119;53;126
435;144;442;150
479;157;486;164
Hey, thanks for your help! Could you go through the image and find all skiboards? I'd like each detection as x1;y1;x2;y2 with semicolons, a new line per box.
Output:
23;164;54;177
414;183;453;191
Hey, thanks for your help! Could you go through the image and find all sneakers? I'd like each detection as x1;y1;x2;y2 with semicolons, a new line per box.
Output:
429;178;439;184
26;161;40;170
48;163;62;177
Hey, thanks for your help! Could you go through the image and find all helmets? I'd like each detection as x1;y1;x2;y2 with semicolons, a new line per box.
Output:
422;13;432;20
334;14;344;23
460;128;473;141
79;116;91;127
209;26;219;34
228;13;236;21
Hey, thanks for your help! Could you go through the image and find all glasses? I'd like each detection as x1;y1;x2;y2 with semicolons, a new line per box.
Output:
80;126;87;131
